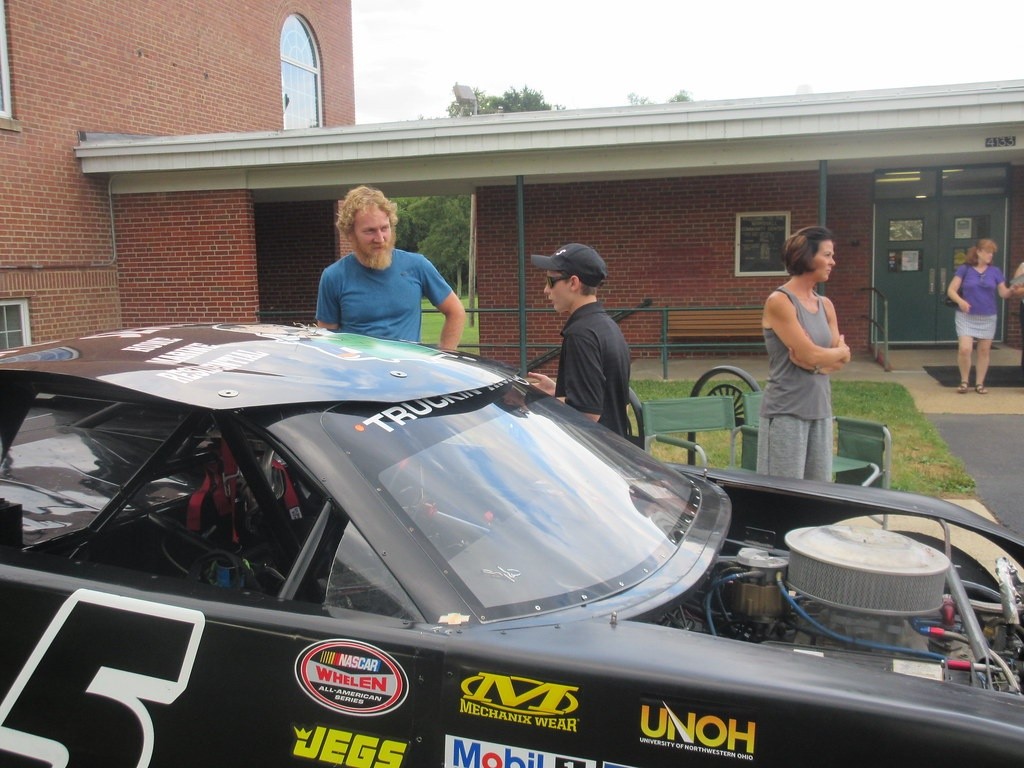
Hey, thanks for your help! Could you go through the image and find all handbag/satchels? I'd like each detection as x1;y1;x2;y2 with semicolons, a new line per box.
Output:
941;263;968;306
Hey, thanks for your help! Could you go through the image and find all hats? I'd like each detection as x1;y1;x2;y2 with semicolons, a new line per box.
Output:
531;243;607;286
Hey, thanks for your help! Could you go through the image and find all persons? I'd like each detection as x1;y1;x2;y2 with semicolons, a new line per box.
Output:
946;239;1024;394
315;185;467;351
761;226;852;483
521;243;632;438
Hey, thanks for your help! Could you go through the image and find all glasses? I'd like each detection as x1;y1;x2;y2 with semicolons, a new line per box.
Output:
546;275;581;288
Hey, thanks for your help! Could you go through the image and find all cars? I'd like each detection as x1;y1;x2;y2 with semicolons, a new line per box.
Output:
0;322;1022;767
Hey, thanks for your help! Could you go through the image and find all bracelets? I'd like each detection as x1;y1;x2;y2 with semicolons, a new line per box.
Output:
809;365;821;374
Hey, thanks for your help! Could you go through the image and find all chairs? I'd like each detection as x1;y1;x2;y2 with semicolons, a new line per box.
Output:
642;391;892;530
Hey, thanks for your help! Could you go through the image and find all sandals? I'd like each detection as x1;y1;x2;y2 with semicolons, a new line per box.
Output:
957;382;970;393
975;384;987;394
